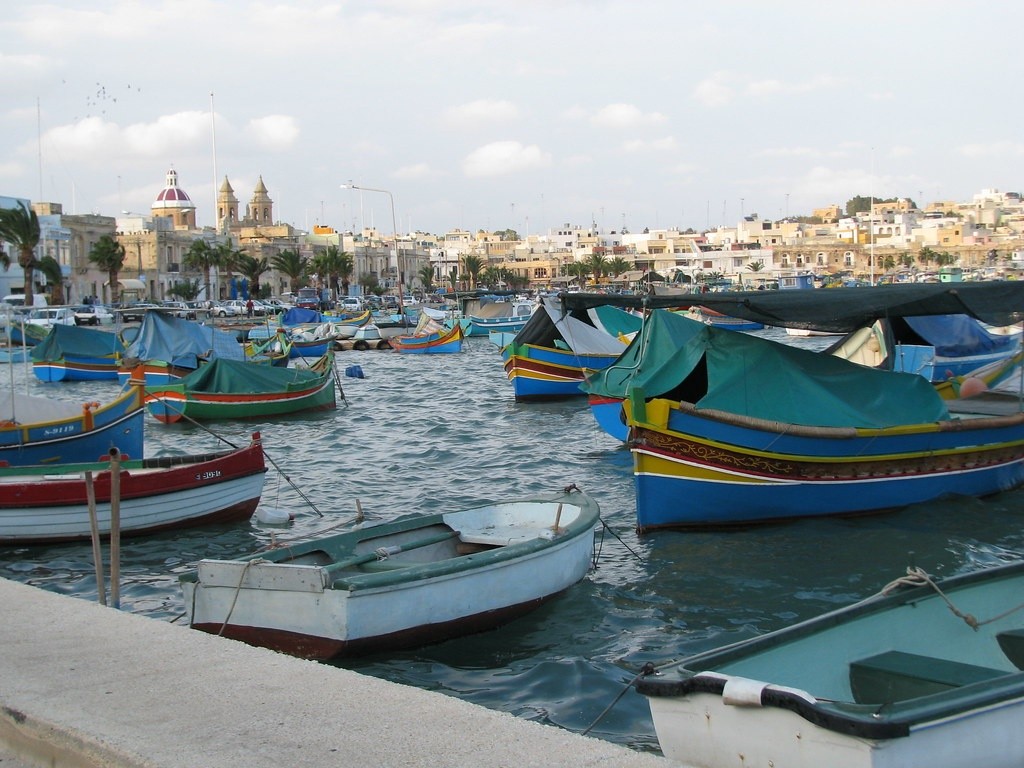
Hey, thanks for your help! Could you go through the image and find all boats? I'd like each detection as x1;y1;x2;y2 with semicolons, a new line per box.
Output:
1;361;151;474
190;480;600;668
630;558;1023;768
144;339;342;423
0;431;270;546
1;272;1023;536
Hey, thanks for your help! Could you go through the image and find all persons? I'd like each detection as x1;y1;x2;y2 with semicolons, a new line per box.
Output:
647;284;655;296
82;296;100;306
246;299;254;319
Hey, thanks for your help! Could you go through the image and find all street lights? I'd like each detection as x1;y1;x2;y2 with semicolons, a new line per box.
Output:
338;180;407;333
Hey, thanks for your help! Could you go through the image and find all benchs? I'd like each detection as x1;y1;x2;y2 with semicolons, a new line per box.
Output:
850;650;1012;704
444;502;581;546
341;560;426;573
